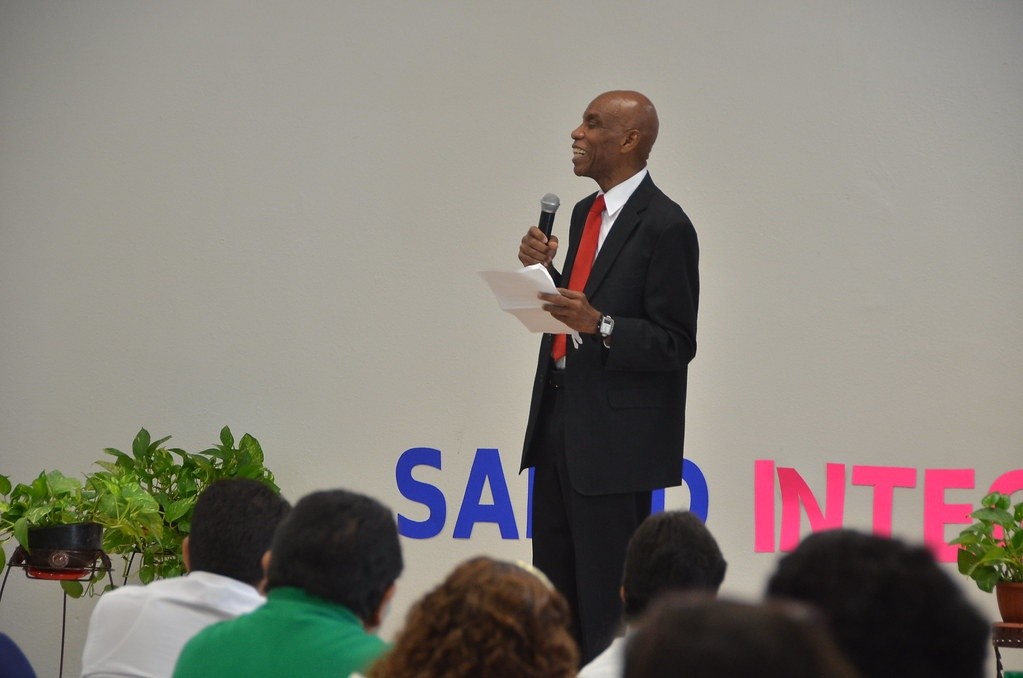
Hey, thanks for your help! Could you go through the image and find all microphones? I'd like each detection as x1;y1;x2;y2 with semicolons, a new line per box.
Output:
537;193;561;246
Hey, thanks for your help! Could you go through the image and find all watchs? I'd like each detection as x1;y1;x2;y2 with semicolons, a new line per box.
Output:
595;311;615;342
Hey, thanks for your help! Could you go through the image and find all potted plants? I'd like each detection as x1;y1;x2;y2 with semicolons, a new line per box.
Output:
0;427;282;598
948;490;1023;624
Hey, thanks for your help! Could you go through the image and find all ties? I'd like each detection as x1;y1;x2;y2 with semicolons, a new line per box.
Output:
553;194;606;362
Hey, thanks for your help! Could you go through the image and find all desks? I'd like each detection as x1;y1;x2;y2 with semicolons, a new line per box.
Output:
991;622;1023;678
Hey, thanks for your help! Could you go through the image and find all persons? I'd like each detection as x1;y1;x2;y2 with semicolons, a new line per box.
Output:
765;528;991;678
172;489;405;677
81;477;291;678
363;556;579;678
518;90;701;672
576;509;727;678
623;598;857;678
